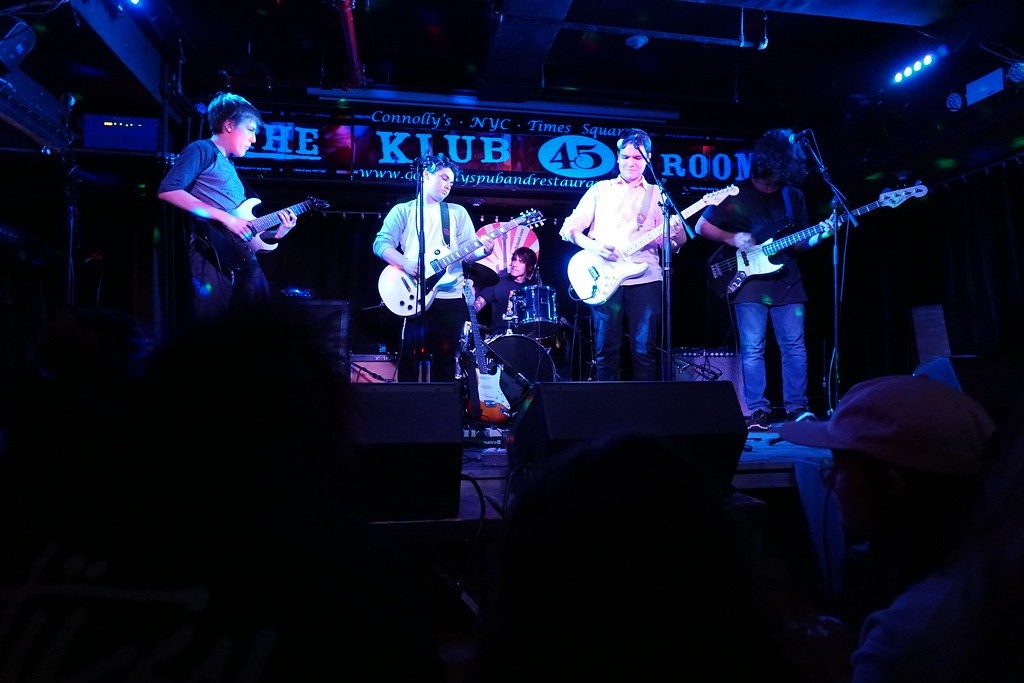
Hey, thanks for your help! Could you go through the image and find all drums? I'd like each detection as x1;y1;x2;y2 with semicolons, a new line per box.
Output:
453;320;494;380
507;285;562;341
482;332;557;415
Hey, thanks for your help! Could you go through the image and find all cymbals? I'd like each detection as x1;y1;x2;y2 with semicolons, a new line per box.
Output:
461;258;500;288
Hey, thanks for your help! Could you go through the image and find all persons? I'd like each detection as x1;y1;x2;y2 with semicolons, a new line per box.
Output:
856;165;1024;683
1;307;448;683
158;94;298;312
372;158;494;382
695;130;842;430
771;373;996;628
472;436;858;683
474;247;543;341
559;129;687;380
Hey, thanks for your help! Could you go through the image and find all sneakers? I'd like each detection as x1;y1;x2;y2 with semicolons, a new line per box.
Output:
746;410;771;430
790;408;818;422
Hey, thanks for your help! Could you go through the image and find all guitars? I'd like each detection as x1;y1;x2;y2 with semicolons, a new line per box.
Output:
702;180;927;298
464;277;512;423
197;195;330;280
566;184;739;307
378;210;546;319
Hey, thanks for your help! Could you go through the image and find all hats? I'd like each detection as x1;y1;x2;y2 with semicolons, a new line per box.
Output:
778;373;1004;473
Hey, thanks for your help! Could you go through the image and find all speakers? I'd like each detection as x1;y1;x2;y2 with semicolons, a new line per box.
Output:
652;348;752;419
350;353;399;383
305;301;353;364
338;380;462;530
910;352;988;400
509;379;749;522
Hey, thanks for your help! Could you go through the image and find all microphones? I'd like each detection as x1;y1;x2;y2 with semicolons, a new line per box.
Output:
386;379;396;382
534;267;540;284
617;133;640;149
425;159;436;172
709;373;722;381
788;129;811;144
